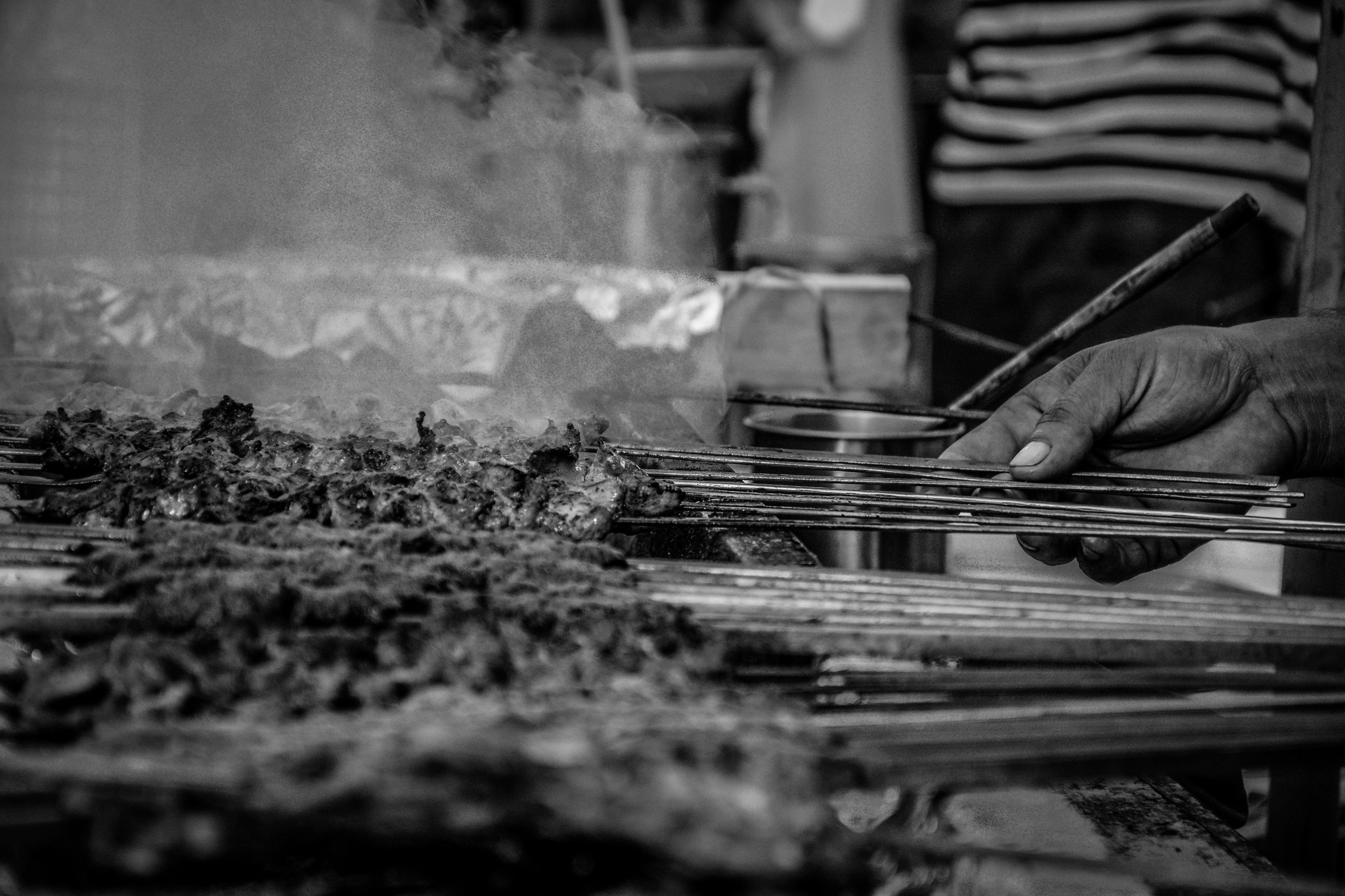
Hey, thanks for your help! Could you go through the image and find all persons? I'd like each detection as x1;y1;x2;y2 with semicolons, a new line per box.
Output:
926;0;1333;444
922;262;1343;590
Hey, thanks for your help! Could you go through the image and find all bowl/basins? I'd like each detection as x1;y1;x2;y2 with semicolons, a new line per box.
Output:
741;406;966;576
